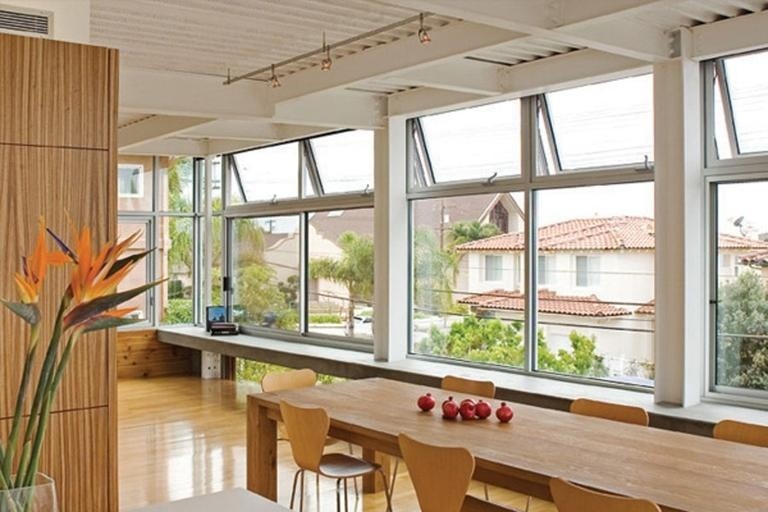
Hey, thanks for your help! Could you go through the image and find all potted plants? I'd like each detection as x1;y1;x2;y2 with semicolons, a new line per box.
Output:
0;206;174;511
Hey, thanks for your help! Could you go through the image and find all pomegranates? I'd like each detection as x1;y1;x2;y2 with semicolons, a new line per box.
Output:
417;393;513;423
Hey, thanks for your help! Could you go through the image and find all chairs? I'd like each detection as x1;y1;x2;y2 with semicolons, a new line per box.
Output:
714;420;768;448
570;398;650;426
280;399;393;512
260;368;354;454
549;477;662;512
397;433;518;512
388;377;495;510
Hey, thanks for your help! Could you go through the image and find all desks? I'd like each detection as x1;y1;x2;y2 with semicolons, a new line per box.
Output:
244;377;768;512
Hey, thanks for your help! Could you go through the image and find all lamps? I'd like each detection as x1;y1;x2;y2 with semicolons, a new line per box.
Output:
416;10;432;47
321;45;332;72
